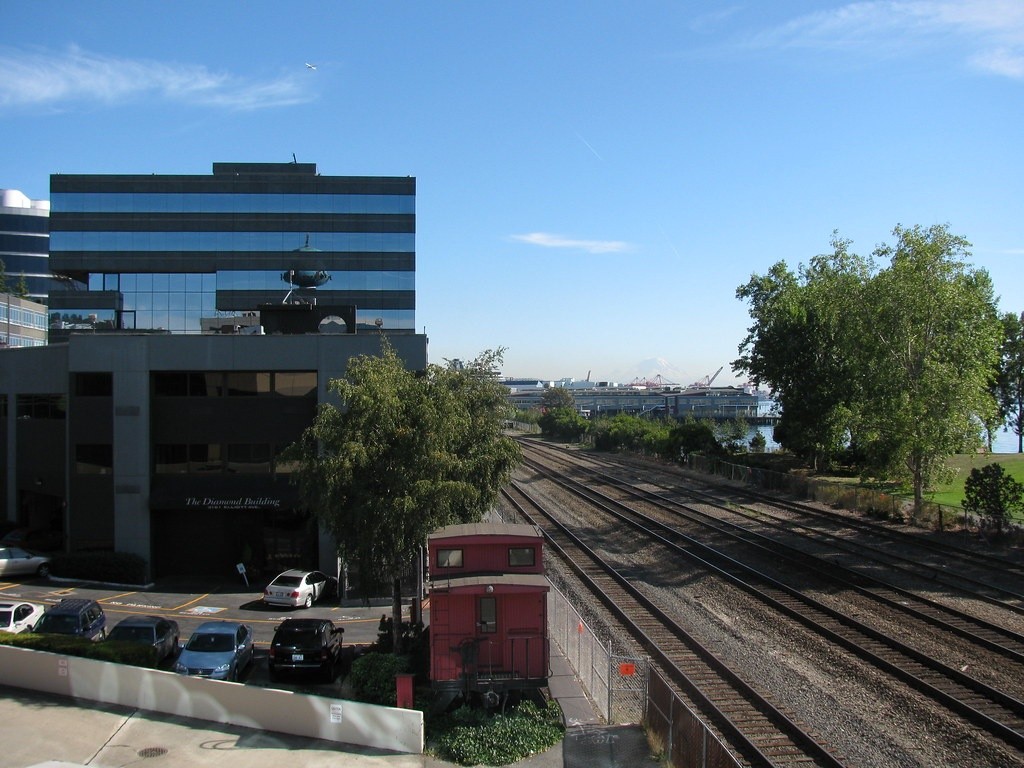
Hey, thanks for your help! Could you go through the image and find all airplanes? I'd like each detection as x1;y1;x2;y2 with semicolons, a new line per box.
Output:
304;62;318;70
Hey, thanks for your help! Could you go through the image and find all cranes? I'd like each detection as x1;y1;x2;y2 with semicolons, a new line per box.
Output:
699;367;724;387
631;373;680;391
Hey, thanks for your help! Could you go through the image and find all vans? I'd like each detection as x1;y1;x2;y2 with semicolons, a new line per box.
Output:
27;599;108;646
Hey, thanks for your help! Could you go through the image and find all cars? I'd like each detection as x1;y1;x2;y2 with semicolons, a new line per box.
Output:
176;621;254;683
268;619;345;684
264;569;338;609
0;602;45;635
105;615;180;667
0;547;52;578
